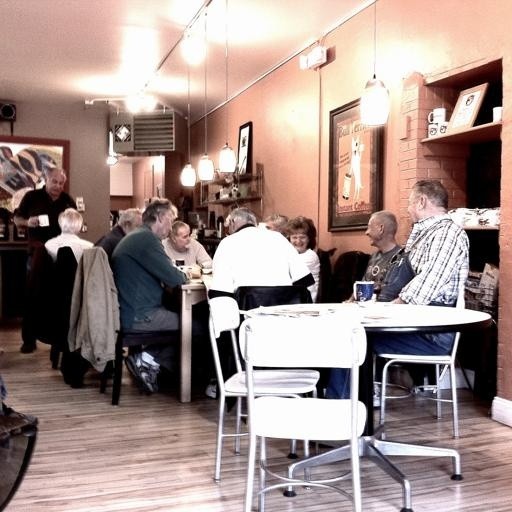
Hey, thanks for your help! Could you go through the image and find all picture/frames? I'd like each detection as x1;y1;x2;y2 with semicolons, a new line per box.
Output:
0;136;70;218
327;99;383;232
445;83;488;134
238;122;252;176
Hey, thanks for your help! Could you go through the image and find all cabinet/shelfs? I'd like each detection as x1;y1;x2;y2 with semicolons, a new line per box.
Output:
189;165;264;244
422;53;503;426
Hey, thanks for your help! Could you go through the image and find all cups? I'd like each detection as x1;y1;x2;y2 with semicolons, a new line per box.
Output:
353;280;375;302
37;214;50;227
201;261;213;276
427;123;438;138
239;183;249;198
427;108;447;124
176;260;185;267
185;255;197;267
437;121;449;137
180;266;193;284
219;189;230;200
492;107;503;123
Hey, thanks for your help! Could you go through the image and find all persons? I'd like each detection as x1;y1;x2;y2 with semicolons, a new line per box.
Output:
161;221;214;270
284;215;332;305
95;207;143;261
109;197;194;395
341;210;405;303
323;179;471;398
203;206;316;398
11;166;78;353
44;208;96;266
266;214;290;241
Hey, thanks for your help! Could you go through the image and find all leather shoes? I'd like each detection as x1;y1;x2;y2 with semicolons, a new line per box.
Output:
19;340;38;353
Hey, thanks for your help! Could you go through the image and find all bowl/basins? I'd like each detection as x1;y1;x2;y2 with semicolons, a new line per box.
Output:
204;229;216;238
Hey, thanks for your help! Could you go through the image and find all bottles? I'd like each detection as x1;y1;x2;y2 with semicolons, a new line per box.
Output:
216;216;224;239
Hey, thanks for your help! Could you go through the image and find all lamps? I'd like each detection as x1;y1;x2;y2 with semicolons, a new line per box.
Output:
218;1;236;174
359;0;391;129
180;33;197;188
198;11;214;181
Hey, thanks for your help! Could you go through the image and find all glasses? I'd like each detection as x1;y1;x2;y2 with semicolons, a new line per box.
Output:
388;247;407;263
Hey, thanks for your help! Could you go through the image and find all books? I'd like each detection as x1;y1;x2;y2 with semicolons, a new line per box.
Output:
464;261;499;308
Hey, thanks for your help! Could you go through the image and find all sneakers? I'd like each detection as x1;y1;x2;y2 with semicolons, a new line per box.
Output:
123;352;155;396
148;358;161;396
205;378;218;399
